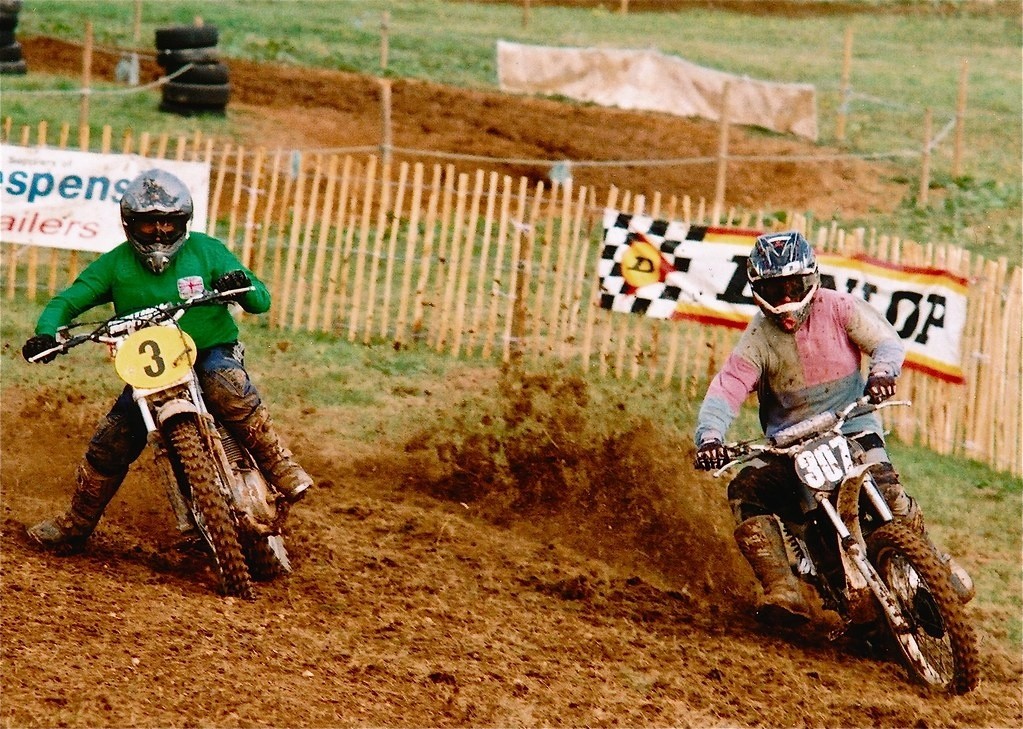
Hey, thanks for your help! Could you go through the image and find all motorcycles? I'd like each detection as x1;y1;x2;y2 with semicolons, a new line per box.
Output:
691;394;981;698
26;287;297;604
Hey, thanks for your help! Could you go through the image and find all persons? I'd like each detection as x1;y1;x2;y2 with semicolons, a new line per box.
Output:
24;170;314;546
694;232;975;623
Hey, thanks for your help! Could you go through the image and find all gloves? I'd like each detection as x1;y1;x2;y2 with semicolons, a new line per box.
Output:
212;269;249;301
695;439;726;470
864;376;897;404
22;335;58;364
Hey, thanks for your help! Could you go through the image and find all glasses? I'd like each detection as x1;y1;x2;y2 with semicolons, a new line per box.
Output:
130;213;182;243
755;277;805;302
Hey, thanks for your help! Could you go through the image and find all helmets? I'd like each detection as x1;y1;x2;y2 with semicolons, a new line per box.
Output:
120;169;193;274
746;231;821;334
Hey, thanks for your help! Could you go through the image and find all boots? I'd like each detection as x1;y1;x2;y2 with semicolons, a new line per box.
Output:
221;397;314;503
732;514;811;628
898;496;977;602
28;454;129;553
